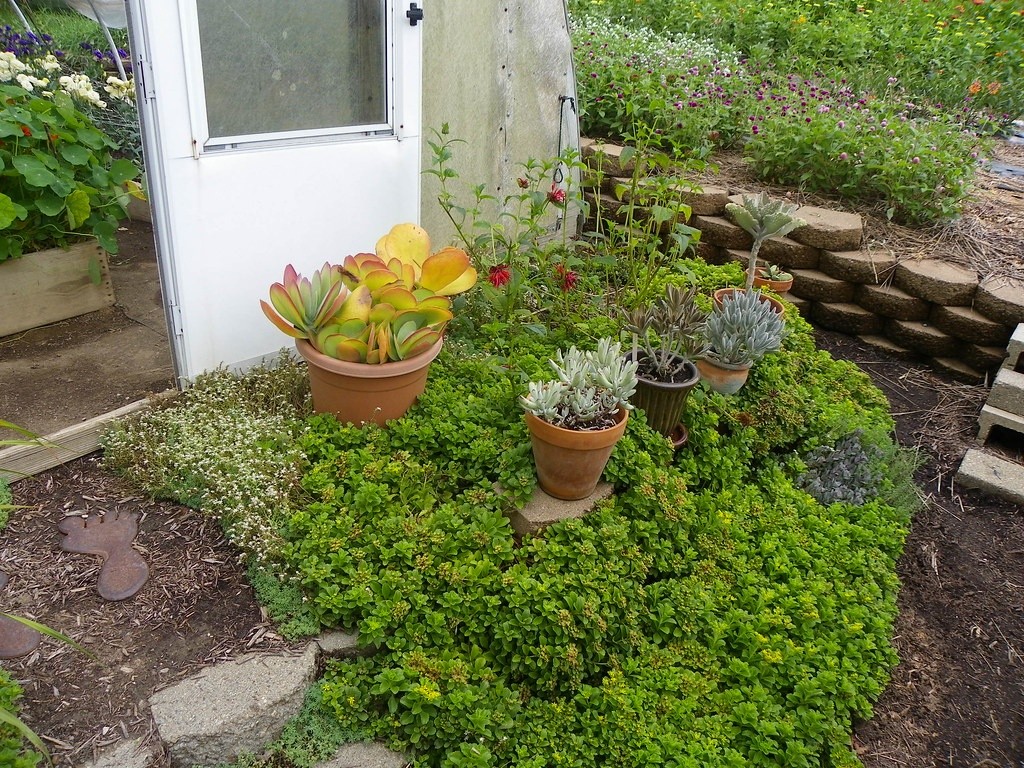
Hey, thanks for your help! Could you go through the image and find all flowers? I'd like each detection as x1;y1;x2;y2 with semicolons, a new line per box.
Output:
418;118;580;325
0;25;133;76
0;50;135;122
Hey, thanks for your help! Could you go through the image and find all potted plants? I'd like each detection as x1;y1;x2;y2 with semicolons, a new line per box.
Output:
0;85;147;337
697;288;789;395
520;336;639;501
261;223;477;427
714;192;808;321
746;261;793;299
620;283;712;439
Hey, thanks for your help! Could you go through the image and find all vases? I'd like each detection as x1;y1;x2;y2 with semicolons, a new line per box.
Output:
666;422;688;457
